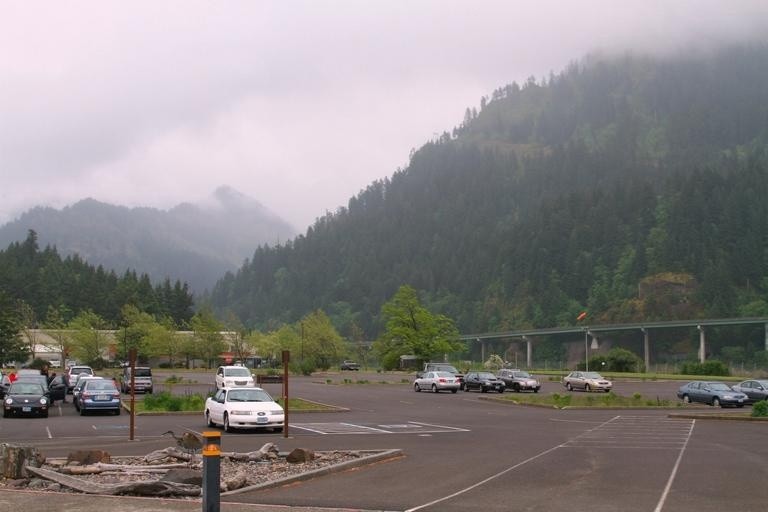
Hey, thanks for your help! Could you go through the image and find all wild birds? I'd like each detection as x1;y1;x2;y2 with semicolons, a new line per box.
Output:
161;430;202;464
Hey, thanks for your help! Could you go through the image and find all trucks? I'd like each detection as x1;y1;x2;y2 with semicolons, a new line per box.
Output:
243;356;265;368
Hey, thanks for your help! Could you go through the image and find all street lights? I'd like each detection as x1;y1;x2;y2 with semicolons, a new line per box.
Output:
119;319;130;375
299;321;304;361
582;326;590;371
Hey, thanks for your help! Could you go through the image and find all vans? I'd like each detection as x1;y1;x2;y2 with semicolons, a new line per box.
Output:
214;365;256;390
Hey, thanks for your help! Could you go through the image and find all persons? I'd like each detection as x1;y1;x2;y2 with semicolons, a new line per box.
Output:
0;366;70;404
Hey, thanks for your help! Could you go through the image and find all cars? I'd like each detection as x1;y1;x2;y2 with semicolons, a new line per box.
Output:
0;361;121;418
676;381;749;408
204;387;285;434
732;378;767;406
233;361;244;367
341;359;360;370
563;371;612;392
413;371;460;394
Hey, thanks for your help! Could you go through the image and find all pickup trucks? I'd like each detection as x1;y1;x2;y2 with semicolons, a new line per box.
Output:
119;365;153;393
417;364;465;390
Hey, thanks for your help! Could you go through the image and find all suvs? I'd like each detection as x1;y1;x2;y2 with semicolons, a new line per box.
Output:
497;368;540;393
463;371;506;393
